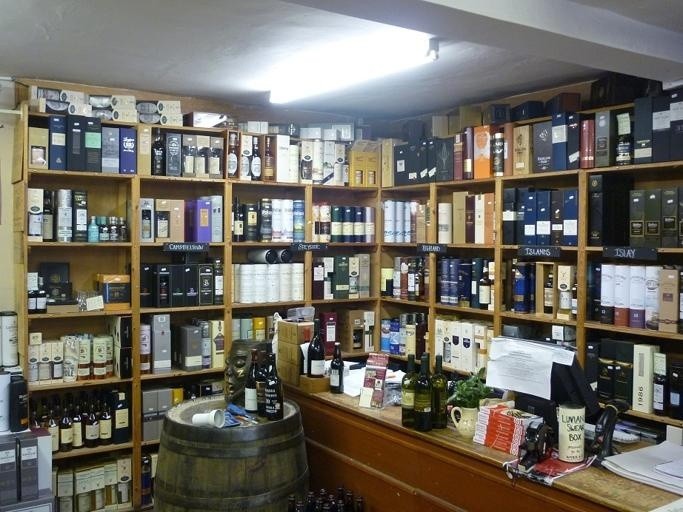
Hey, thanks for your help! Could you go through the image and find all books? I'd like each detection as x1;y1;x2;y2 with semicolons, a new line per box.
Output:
472;405;543;457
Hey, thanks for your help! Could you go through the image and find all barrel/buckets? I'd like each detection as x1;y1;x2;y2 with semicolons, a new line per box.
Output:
154;392;311;512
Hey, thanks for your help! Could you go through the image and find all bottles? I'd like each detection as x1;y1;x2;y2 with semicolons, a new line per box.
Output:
614;119;632;166
477;259;492;310
306;315;344;394
541;269;577;320
242;347;283;421
407;257;425;303
151;128;276;188
88;215;128;243
26;187;54;314
231;196;245;243
399;349;451;434
653;371;669;416
27;387;133;454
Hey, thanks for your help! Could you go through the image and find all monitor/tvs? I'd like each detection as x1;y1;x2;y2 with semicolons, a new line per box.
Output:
491;335;604;424
